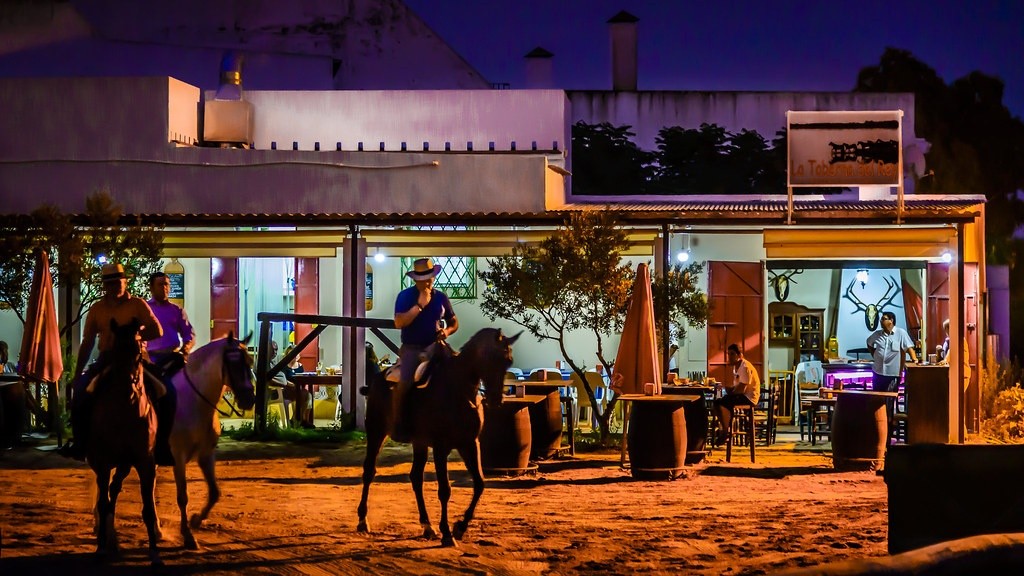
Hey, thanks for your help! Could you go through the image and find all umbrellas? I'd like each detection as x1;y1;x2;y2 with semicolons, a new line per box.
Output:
609;263;663;465
17;248;64;427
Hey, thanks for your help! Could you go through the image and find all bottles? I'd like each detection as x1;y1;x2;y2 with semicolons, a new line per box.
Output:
688;371;705;384
799;316;820;363
830;375;834;384
818;387;833;399
839;379;843;390
823;335;838;360
928;354;937;365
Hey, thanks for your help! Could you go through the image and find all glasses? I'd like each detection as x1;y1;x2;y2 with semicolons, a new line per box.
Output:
882;316;890;320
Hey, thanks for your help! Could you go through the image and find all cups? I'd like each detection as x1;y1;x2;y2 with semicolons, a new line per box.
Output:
708;377;715;386
316;362;336;376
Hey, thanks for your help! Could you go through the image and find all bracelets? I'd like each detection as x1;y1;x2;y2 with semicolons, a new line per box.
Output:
417;303;423;311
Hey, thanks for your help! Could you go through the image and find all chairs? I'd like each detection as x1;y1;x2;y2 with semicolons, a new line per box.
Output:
750;374;780;447
499;366;608;435
791;358;907;447
249;369;290;429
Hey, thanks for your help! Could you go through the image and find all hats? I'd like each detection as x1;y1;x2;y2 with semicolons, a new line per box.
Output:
94;264;134;283
406;258;442;280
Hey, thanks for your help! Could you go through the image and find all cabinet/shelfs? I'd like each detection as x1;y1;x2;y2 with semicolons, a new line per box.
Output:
768;302;826;361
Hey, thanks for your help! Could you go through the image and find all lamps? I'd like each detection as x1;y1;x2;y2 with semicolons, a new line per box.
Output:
856;269;869;289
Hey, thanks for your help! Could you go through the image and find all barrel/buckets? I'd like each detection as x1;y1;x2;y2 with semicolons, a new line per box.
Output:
831;393;888;472
663;389;708;464
628;401;687;480
479;403;532;476
524;387;563;460
0;380;25;452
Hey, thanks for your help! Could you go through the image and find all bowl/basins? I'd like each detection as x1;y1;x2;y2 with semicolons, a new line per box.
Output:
673;378;689;386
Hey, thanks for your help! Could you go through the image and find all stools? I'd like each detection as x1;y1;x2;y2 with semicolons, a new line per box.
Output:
713;405;753;465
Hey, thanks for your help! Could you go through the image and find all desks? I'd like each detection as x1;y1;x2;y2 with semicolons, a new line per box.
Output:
615;393;702;479
503;378;576;460
823;389;898;474
481;393;546;479
659;377;723;451
290;370;343;430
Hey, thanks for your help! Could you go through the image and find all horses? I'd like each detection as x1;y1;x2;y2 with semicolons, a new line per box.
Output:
357;328;525;547
69;329;256;576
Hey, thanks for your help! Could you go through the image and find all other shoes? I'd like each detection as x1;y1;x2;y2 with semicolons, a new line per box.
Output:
392;426;408;442
158;444;175;467
715;430;728;445
290;420;315;429
56;443;86;461
41;409;55;425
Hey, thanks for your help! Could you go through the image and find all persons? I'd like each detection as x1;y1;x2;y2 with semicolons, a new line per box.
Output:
715;344;760;447
270;341;316;429
386;258;459;440
866;311;919;414
941;319;971;440
365;342;383;386
0;341;48;427
57;263;196;462
